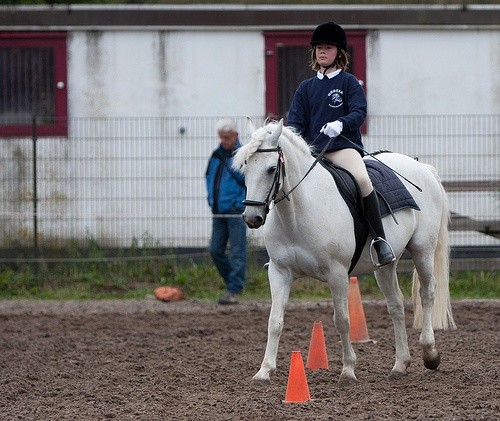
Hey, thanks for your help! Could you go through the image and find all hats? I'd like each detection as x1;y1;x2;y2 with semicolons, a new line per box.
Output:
310;22;347;47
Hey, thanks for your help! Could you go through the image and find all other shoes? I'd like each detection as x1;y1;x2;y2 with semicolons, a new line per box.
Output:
219;292;240;303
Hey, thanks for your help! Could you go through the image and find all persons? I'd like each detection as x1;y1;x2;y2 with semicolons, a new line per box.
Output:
203;122;250;306
285;21;397;268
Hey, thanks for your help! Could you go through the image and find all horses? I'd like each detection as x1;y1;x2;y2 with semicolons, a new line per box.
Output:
230;118;454;387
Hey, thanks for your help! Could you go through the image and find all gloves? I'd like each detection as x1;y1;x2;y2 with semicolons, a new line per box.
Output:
320;120;344;139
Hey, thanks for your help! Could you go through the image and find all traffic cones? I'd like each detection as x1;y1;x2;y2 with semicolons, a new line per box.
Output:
340;277;371;344
306;322;329;370
281;350;313;404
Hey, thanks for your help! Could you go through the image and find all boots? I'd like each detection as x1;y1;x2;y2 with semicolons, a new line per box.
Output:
361;190;393;264
